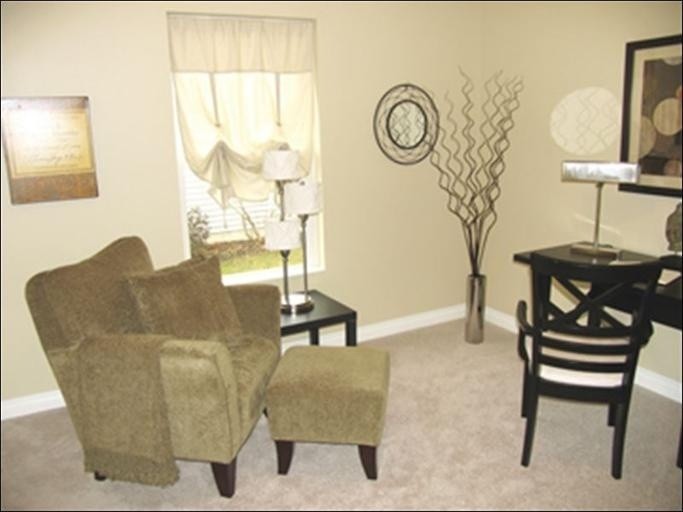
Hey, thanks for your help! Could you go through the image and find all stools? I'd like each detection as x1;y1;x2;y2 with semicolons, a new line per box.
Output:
263;342;393;482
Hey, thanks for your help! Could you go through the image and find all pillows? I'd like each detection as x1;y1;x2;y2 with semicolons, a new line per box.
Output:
152;249;205;278
119;255;244;350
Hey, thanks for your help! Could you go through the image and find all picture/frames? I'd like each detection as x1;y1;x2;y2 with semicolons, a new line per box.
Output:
609;32;682;199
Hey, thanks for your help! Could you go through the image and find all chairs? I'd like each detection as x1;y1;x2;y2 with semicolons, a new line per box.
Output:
22;237;286;499
505;249;663;482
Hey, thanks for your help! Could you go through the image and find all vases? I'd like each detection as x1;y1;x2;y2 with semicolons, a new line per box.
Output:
462;273;488;345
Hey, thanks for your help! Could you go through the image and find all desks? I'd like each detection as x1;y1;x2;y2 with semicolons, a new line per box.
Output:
513;240;680;471
273;287;359;349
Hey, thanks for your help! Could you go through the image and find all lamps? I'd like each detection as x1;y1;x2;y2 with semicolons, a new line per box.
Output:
261;145;318;316
557;158;640;263
